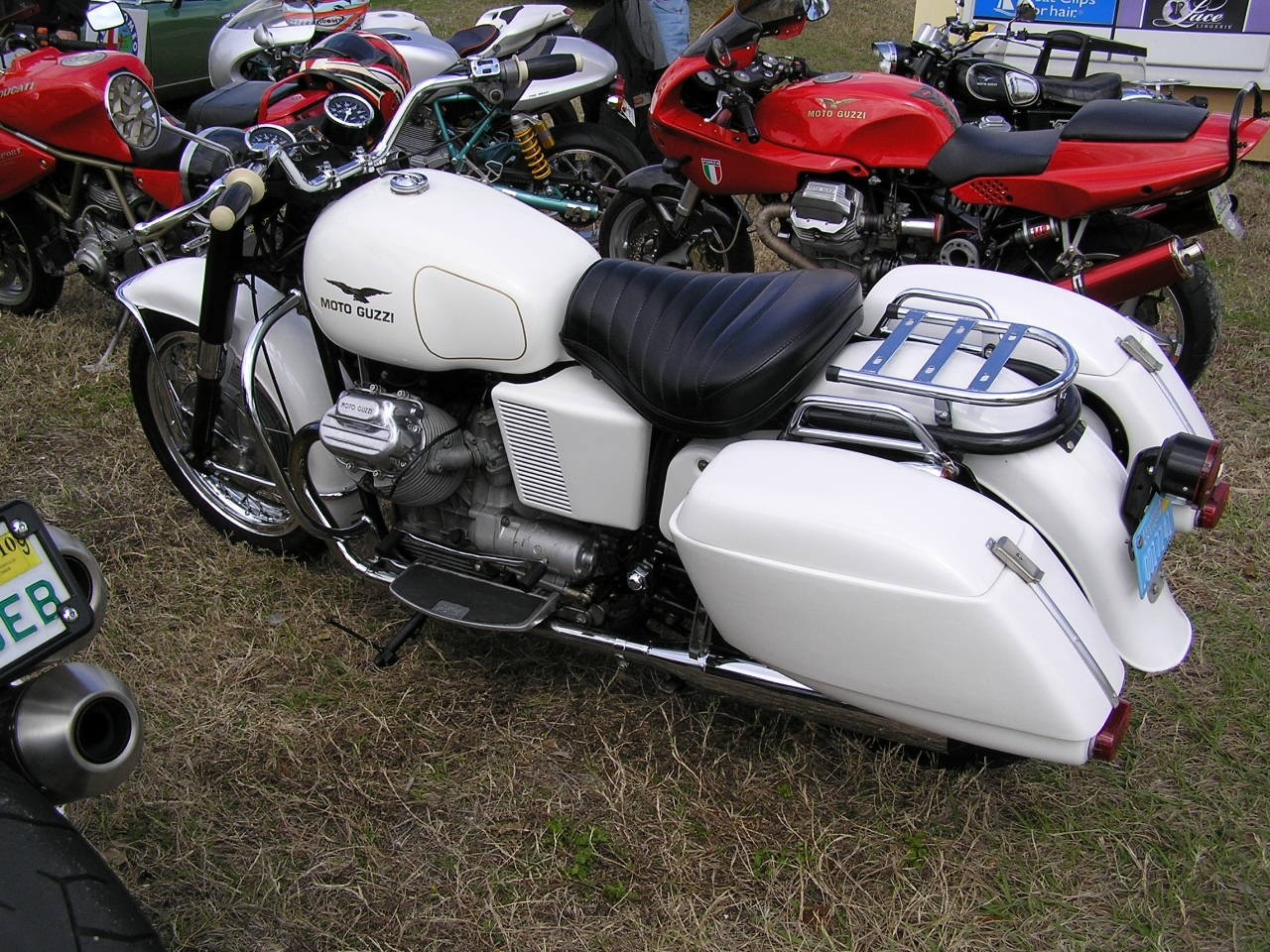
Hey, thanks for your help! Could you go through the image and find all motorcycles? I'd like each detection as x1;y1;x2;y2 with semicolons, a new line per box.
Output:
861;0;1206;133
102;54;1232;768
1;499;169;952
598;0;1269;392
0;0;649;320
